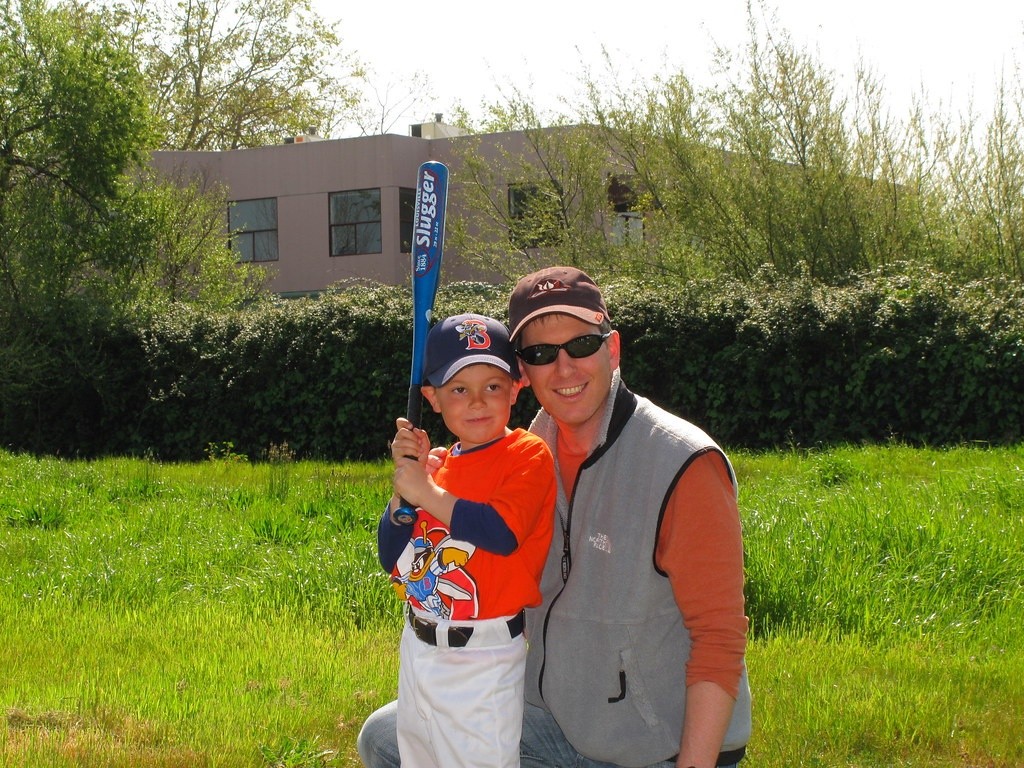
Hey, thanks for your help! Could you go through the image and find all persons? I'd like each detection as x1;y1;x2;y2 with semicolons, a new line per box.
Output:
377;314;556;768
356;266;753;768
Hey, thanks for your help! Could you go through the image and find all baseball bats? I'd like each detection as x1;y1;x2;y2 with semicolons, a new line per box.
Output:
393;162;450;525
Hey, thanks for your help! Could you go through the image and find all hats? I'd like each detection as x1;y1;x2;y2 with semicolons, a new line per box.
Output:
508;265;611;342
423;312;516;388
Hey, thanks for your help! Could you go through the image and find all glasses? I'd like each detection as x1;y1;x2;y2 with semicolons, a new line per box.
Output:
514;331;610;365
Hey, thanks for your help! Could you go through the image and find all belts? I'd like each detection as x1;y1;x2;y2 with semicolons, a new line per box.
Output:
409;609;525;648
